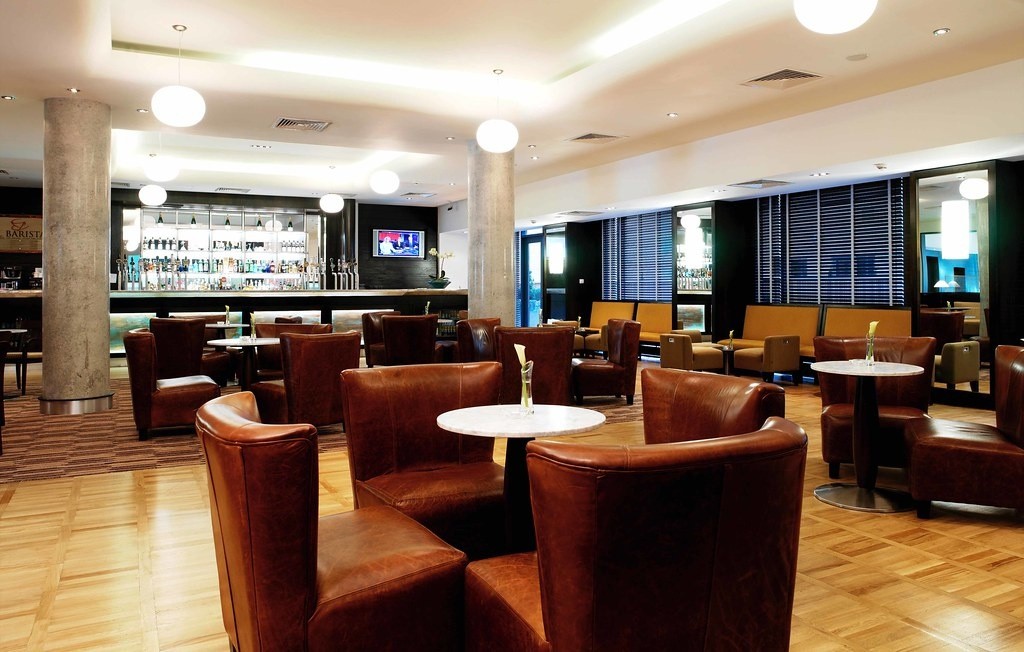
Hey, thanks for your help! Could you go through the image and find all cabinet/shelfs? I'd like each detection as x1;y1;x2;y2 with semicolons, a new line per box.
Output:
120;205;328;290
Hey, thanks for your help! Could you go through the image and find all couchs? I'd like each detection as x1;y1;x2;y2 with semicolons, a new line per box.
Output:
363;310;502;368
641;369;784;445
660;334;724;373
494;326;574;405
585;325;608;359
195;392;468;652
955;301;981;339
553;321;585;356
465;416;808;652
812;335;1024;519
922;310;965;355
340;361;505;553
571;318;641;405
734;335;801;386
122;314;362;442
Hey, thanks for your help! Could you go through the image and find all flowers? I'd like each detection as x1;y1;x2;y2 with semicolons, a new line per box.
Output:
428;248;454;279
577;316;581;327
514;343;530;408
250;313;255;336
426;301;430;312
867;321;880;360
540;308;542;326
947;301;951;307
225;305;229;320
730;330;734;347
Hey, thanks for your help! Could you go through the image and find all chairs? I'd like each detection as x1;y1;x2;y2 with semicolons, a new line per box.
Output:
5;325;43;390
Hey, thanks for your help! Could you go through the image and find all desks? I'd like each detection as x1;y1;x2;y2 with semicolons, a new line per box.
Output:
207;337;281;392
811;359;924;512
205;324;250;340
0;328;27;396
436;403;606;556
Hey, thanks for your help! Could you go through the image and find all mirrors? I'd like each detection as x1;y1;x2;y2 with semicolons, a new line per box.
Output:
544;225;566;320
911;160;991;394
674;207;712;342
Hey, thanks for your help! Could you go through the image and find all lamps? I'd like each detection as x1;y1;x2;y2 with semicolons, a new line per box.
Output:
150;25;206;127
139;185;166;206
476;69;518;152
320;194;344;214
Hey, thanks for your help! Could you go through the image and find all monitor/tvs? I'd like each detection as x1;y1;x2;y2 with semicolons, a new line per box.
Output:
373;229;425;258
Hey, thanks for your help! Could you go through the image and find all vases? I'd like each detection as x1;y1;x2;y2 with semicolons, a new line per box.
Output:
866;335;875;365
539;316;543;327
251;320;257;341
226;312;230;325
428;279;451;289
578;321;581;330
518;360;535;415
729;335;734;349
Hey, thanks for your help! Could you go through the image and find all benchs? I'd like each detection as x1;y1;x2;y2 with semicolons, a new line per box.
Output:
585;300;673;360
716;302;912;383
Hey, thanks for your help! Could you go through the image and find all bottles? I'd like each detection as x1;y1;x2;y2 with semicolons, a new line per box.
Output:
296;241;300;252
180;239;186;251
240;260;286;272
158;237;163;250
199;259;203;272
287;240;292;252
218;258;223;273
213;257;218;273
172;237;176;250
203;258;208;272
183;256;188;271
116;253;180;292
150;237;155;249
164;238;171;250
292;241;296;252
143;237;148;250
282;241;287;251
300;241;304;252
302;257;359;290
193;259;198;274
